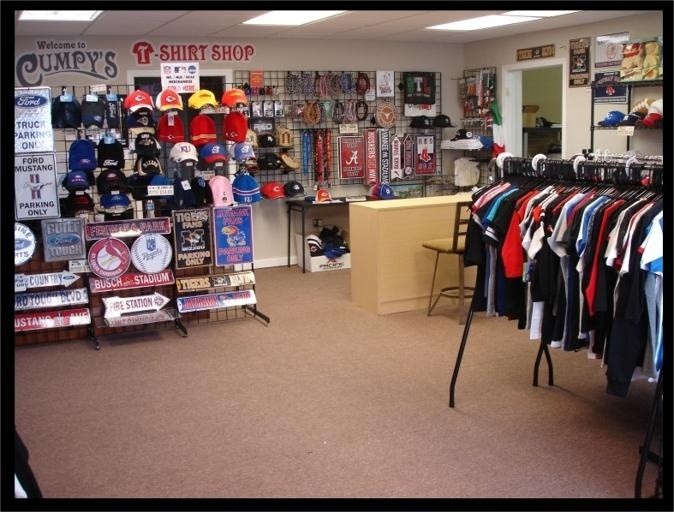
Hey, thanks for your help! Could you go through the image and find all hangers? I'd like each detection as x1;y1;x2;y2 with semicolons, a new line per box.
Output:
492;148;663;201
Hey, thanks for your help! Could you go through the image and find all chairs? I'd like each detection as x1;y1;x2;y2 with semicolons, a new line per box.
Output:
421;201;473;325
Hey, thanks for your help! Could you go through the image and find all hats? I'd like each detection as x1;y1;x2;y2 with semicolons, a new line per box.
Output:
261;127;305;200
308;224;348;259
598;110;659;127
450;129;470;141
410;115;434;129
62;137;131;213
124;88;263;206
315;189;333;203
52;93;105;129
369;183;395;199
434;115;455;128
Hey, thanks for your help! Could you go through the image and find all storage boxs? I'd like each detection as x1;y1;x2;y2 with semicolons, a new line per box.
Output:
521;104;540;128
294;231;351;274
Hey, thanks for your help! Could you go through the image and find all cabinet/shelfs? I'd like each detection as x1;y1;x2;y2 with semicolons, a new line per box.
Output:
587;78;666;133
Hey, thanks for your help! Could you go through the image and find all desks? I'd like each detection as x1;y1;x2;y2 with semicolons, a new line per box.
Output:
283;194;404;274
349;193;479;321
